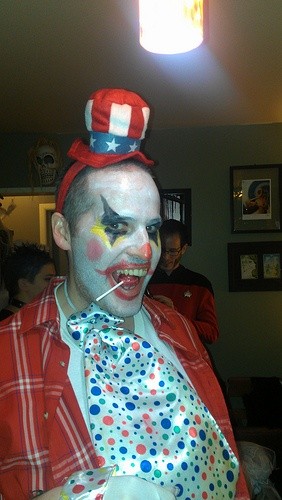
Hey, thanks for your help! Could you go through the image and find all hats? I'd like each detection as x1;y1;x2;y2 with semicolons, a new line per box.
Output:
55;88;155;215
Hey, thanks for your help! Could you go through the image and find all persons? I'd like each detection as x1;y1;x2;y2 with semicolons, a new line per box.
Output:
0;89;250;500
145;219;219;345
0;240;56;322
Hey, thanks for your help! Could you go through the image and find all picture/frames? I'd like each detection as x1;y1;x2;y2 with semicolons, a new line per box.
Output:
228;240;282;292
228;164;282;233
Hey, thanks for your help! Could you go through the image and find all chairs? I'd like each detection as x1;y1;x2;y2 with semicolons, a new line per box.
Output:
226;377;282;456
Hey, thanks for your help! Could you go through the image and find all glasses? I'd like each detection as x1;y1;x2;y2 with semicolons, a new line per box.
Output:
161;247;183;256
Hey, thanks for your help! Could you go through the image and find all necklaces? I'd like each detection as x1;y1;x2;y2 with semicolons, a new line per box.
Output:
32;490;46;499
11;298;25;310
